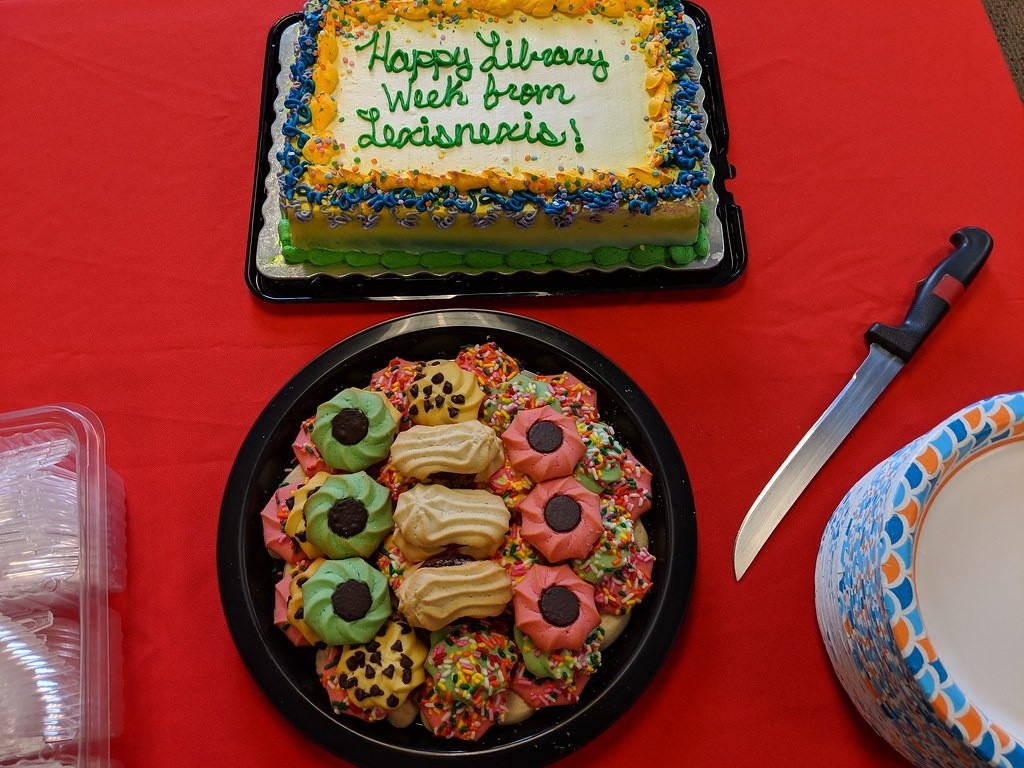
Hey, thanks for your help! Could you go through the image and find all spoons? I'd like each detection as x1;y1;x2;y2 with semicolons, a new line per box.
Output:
0;608;80;768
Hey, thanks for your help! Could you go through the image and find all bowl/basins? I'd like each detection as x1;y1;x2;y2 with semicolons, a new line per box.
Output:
0;402;128;768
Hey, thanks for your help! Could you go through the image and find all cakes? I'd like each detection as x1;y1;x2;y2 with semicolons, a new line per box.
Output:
276;0;710;266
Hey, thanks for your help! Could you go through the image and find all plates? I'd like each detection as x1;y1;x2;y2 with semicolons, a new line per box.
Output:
815;392;1024;768
217;308;695;768
244;9;747;302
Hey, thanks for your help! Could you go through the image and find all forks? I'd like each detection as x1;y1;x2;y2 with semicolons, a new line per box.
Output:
0;439;79;604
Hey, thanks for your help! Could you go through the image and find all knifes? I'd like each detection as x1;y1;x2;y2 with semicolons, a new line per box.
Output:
733;226;992;581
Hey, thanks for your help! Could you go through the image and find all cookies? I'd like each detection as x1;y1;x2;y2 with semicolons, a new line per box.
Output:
259;343;656;742
